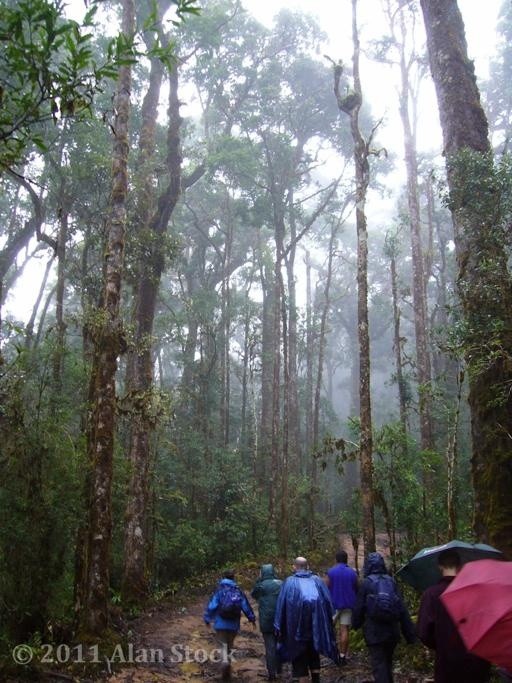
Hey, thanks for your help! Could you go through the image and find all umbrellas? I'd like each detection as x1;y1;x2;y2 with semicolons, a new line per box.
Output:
395;539;504;590
438;558;512;672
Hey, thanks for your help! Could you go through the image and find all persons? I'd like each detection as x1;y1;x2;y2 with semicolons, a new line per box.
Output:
350;552;417;683
273;556;337;683
250;563;285;681
324;549;362;668
415;550;494;683
203;571;257;683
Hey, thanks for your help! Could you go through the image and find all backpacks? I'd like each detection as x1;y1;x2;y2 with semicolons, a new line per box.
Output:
364;572;398;621
217;582;242;620
